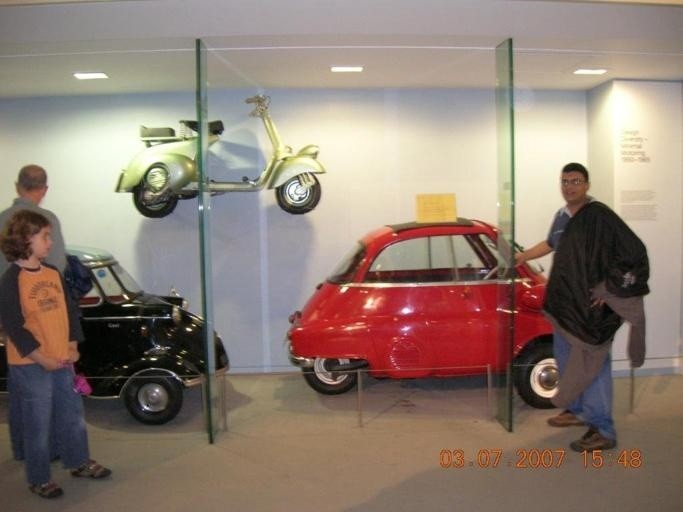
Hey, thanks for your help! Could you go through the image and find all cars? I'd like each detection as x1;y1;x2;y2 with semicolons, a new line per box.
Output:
280;211;561;412
0;245;236;430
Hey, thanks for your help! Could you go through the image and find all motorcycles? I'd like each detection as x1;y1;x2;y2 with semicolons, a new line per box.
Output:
111;89;332;221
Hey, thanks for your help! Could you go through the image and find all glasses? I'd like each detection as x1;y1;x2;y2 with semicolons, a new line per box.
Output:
560;178;587;186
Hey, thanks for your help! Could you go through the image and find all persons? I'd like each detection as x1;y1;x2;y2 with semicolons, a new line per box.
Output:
0;164;73;463
507;163;650;454
0;210;111;499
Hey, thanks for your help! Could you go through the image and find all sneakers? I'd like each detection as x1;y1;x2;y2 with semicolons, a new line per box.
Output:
69;459;110;479
546;410;585;427
28;479;63;499
568;426;616;452
